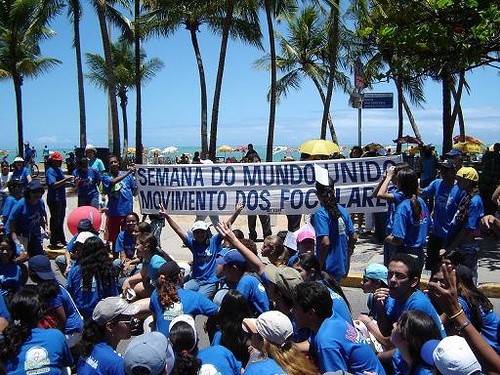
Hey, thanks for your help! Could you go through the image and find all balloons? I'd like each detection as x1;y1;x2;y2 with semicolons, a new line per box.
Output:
67;206;102;235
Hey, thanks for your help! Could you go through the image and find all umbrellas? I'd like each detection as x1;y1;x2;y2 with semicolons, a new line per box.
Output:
217;146;233;151
454;135;485;144
127;148;136;152
394;135;422;150
453;143;483;152
298;140;339;156
234;145;249;150
405;145;421;153
162;146;177;153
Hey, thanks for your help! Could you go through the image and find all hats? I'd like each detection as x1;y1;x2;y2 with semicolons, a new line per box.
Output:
447;149;462;155
191;220;210;232
265;263;304;291
437;157;462;169
29;255;56;280
49;152;63;160
93;297;141;325
14;157;25;162
124;331;175;375
456;167;479;181
363;264;388;285
216;249;246;264
296;231;315;242
85;144;97;152
25;180;45;192
422;336;483;375
169;314;197;352
242;311;293;344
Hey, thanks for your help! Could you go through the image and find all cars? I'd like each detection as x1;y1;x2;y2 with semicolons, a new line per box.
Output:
66;147;112;173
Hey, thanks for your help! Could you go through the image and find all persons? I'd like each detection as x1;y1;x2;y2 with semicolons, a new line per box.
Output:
0;136;500;375
46;152;73;247
290;282;386;375
314;176;357;284
29;256;84;348
157;202;246;300
244;310;322;375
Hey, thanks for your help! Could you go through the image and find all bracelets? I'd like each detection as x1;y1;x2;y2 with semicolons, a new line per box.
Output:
458;320;471;330
448;308;463;320
364;319;372;325
